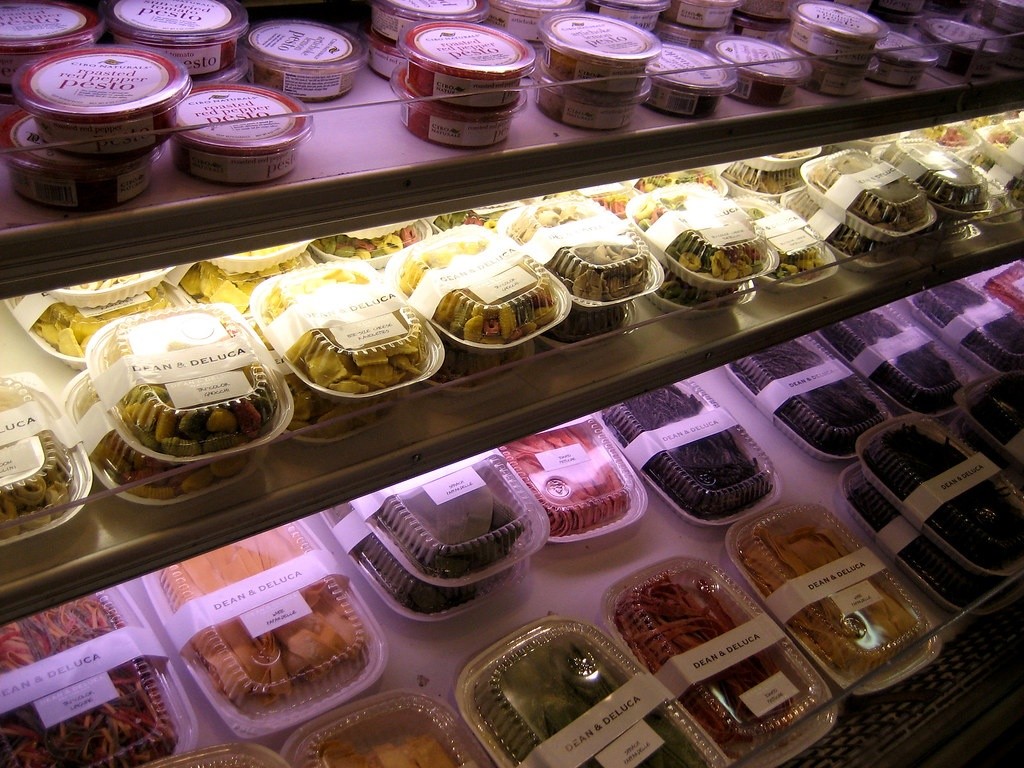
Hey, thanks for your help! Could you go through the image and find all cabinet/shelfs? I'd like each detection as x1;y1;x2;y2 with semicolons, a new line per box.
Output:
0;32;1024;768
